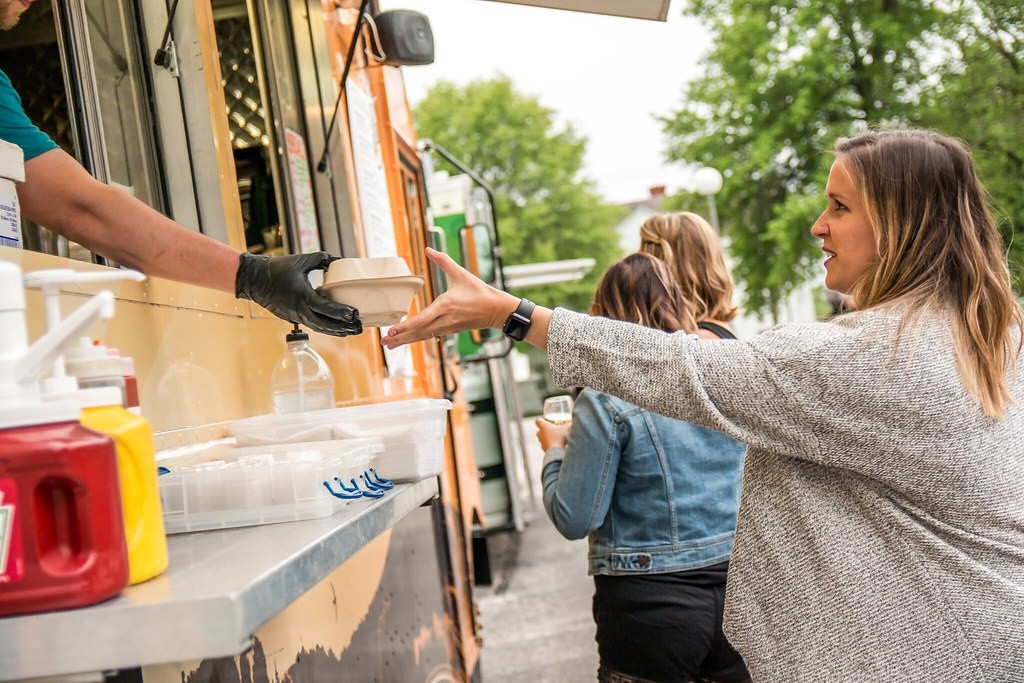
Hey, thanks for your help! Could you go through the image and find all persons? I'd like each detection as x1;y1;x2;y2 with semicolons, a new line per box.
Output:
639;211;746;342
0;0;363;336
536;253;759;683
380;130;1024;683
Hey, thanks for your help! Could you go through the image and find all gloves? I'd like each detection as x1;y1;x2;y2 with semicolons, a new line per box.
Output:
234;251;364;338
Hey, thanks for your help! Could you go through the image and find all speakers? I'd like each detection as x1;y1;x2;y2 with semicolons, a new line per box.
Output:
368;9;435;68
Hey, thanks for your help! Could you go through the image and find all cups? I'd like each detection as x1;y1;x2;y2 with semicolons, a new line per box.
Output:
544;395;576;451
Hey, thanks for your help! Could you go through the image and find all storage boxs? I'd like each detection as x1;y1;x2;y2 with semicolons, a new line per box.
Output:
156;397;453;535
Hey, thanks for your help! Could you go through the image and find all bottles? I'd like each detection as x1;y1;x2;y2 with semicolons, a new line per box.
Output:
269;322;335;412
67;337;141;414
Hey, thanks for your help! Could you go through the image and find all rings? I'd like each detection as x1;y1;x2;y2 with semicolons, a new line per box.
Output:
431;333;440;342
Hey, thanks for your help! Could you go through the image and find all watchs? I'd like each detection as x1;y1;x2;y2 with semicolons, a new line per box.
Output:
503;298;535;342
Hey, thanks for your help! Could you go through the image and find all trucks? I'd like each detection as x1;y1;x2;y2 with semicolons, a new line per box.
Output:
414;143;530;584
0;0;512;683
